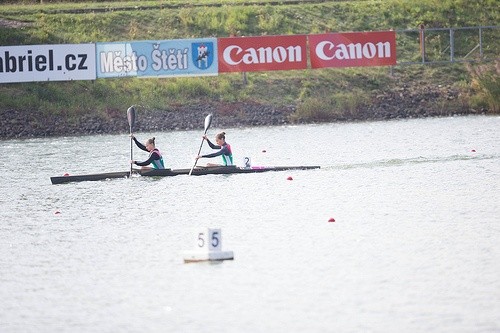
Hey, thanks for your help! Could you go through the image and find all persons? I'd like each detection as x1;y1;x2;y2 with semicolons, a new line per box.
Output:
196;131;236;167
127;135;165;170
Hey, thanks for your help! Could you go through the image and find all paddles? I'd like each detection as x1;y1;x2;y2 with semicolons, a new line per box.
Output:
188;114;213;176
126;107;135;177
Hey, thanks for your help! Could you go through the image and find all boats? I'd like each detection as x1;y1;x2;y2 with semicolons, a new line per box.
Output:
49;163;322;185
